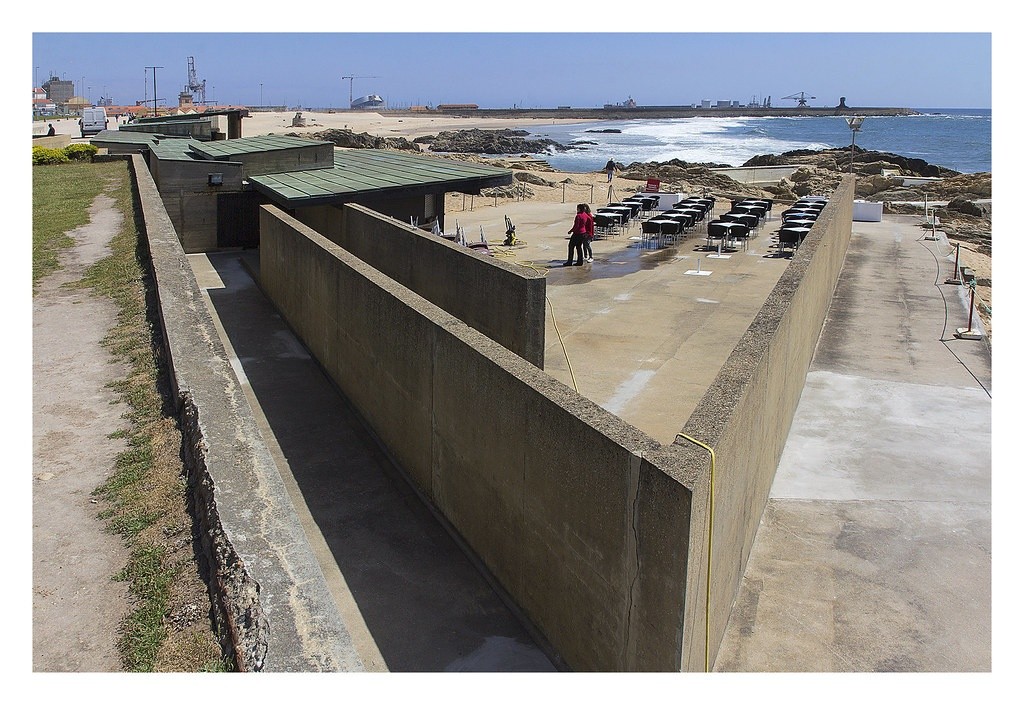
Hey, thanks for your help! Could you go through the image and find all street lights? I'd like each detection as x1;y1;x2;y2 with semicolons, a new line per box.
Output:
146;67;164;117
82;77;85;109
36;66;39;108
847;117;864;172
260;83;263;107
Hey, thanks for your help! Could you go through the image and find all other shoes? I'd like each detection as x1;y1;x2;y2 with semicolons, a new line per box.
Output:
574;263;583;266
587;258;594;263
563;261;572;266
583;257;588;261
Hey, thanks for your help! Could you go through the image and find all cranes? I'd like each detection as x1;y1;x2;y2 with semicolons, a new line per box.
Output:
781;92;817;106
342;73;382;102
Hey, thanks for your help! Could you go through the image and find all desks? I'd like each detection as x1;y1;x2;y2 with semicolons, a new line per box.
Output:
852;200;884;222
593;195;828;257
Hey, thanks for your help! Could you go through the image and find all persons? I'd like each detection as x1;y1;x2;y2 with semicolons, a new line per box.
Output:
605;158;617;183
563;203;594;266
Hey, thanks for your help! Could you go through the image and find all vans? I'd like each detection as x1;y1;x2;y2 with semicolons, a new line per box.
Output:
80;106;109;137
